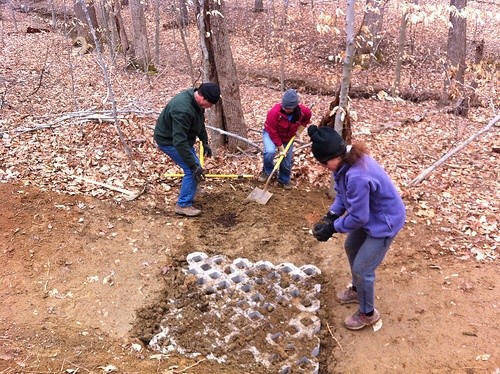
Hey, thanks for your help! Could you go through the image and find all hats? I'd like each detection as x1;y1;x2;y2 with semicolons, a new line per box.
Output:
308;124;346;163
282;89;298;108
201;83;220;104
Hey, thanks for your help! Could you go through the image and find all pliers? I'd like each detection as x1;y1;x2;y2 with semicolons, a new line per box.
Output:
245;134;297;205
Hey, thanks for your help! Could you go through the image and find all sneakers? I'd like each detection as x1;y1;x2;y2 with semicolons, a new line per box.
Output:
342;307;380;330
279;182;292;189
335;287;360;304
258;168;269;181
175;203;202;216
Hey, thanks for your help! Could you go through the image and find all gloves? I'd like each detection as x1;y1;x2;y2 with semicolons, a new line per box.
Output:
312;211;338;242
190;163;206;182
202;141;212;158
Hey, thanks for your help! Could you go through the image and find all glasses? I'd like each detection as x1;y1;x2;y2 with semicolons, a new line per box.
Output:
322;160;328;165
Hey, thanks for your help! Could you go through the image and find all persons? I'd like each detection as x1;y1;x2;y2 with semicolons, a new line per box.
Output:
259;87;313;185
308;125;408;329
154;81;222;218
